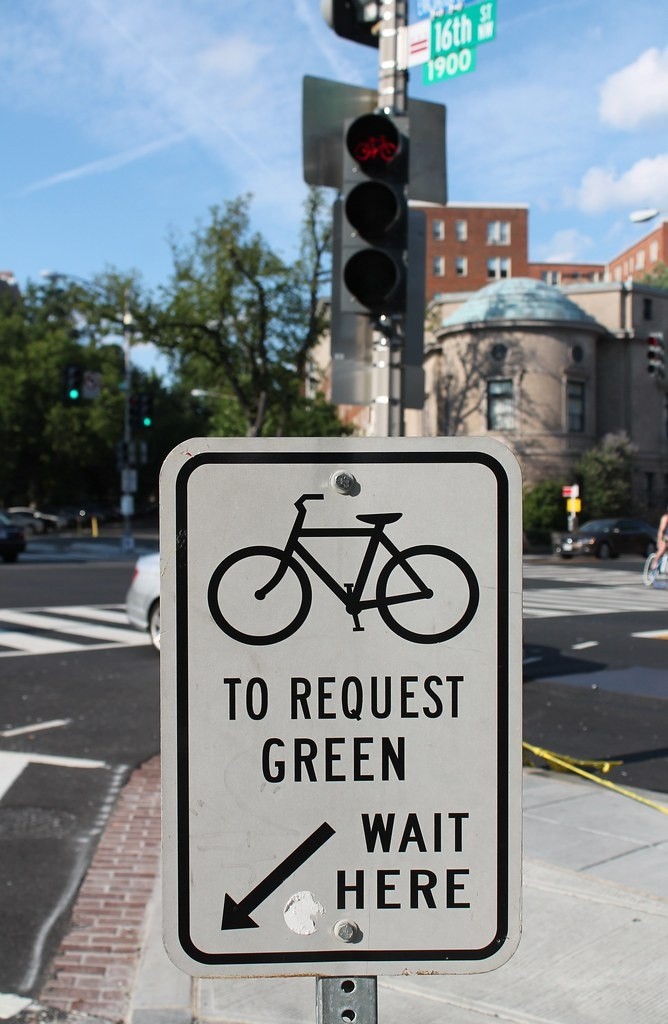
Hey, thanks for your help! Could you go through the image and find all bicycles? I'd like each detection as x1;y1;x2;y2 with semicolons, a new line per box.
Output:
642;544;668;588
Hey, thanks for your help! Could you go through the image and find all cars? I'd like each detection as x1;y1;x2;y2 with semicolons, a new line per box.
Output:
0;505;123;564
126;552;160;653
557;516;659;562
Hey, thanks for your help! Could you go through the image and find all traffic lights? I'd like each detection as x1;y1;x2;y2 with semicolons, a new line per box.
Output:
344;106;415;313
128;392;153;433
62;364;84;406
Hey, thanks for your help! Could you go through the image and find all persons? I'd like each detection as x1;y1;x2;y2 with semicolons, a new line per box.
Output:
651;514;668;570
